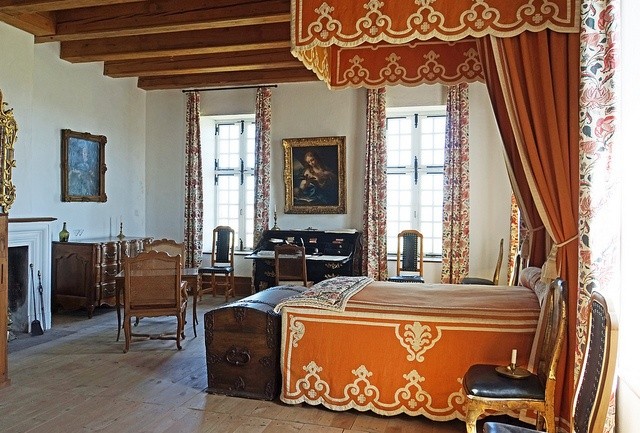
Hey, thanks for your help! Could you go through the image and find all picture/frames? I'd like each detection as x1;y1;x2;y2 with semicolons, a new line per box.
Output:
60;128;107;203
282;136;348;215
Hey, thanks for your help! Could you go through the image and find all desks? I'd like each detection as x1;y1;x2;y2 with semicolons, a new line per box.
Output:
114;269;198;342
244;229;363;292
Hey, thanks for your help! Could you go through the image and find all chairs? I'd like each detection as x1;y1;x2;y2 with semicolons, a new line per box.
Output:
134;238;188;327
274;244;314;288
462;277;569;433
462;239;503;285
198;226;235;302
121;250;185;353
384;230;424;283
480;289;619;433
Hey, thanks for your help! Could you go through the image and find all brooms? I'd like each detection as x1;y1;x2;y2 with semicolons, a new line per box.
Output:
29;264;44;336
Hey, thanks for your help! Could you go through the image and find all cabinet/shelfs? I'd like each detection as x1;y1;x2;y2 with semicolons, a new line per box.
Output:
52;236;152;319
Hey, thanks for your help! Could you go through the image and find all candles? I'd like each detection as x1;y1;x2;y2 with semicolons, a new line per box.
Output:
511;349;518;364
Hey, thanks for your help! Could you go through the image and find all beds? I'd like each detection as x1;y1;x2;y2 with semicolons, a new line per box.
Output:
275;267;549;424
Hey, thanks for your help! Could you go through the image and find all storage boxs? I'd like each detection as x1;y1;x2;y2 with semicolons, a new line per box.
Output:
204;286;305;400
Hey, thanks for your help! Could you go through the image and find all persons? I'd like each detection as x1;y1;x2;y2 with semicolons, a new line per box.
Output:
294;151;338;204
